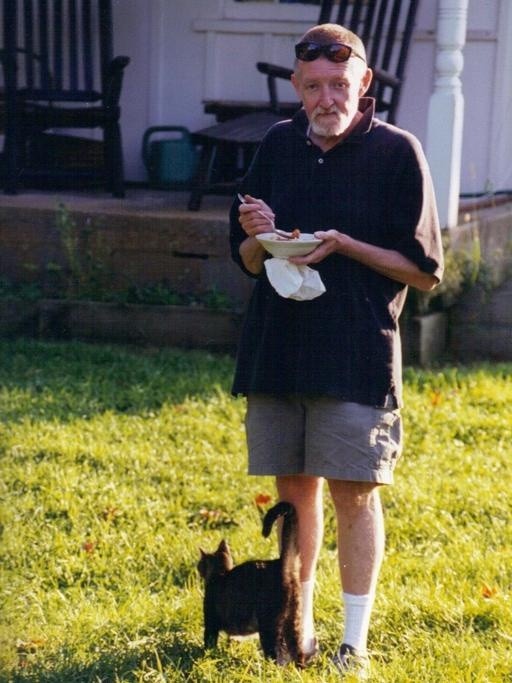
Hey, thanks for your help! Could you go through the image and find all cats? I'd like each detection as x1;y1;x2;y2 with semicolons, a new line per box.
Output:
197;500;309;671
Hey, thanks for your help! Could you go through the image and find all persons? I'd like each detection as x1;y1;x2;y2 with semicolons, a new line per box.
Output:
228;19;445;680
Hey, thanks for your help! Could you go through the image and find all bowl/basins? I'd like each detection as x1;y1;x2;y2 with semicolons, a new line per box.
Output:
257;230;324;258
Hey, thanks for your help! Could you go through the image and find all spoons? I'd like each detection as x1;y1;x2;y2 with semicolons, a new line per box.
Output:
236;193;298;239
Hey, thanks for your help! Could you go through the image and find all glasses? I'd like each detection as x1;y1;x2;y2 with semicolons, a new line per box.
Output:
295;41;366;63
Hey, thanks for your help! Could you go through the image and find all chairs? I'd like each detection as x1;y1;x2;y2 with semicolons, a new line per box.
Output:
185;0;419;212
0;0;130;200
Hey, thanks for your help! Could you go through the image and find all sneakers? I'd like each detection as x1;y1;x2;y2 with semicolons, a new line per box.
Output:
332;644;369;680
